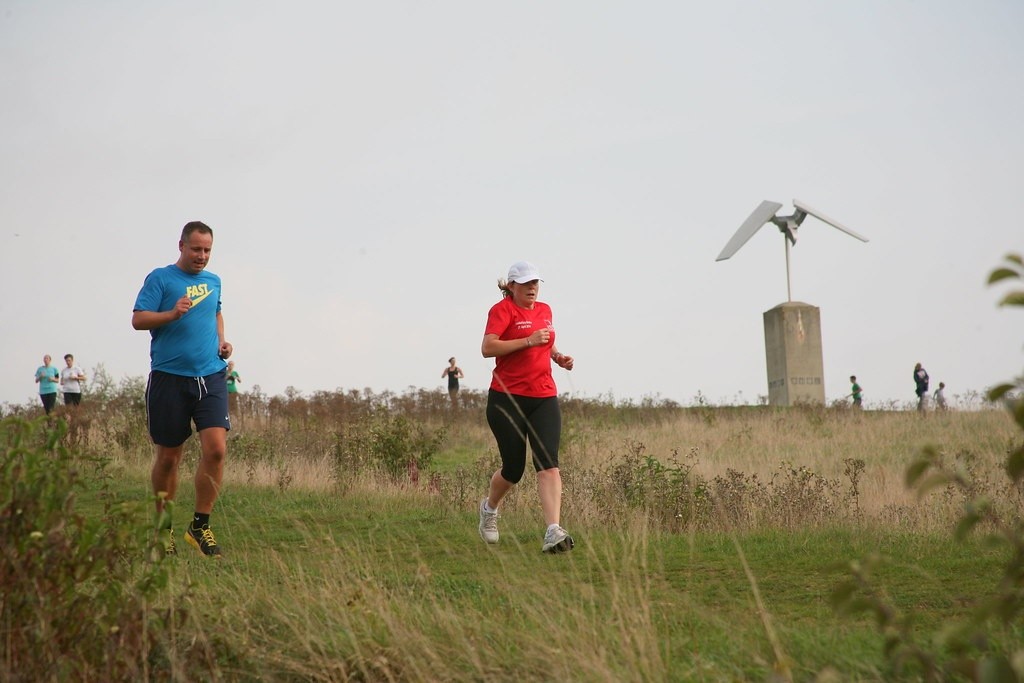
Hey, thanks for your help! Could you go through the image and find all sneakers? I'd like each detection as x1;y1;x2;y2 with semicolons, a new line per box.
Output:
541;525;575;555
479;500;502;544
154;528;178;558
184;520;222;561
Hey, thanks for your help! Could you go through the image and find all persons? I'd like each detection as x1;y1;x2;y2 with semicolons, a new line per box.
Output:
846;375;862;406
442;357;463;400
224;361;241;395
130;221;233;557
33;353;59;416
479;262;575;554
933;382;947;410
61;354;85;407
914;363;930;412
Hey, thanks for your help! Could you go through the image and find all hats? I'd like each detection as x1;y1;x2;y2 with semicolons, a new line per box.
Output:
508;261;545;284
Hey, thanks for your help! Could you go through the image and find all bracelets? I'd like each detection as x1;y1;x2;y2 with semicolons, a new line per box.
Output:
552;352;561;363
526;337;531;346
